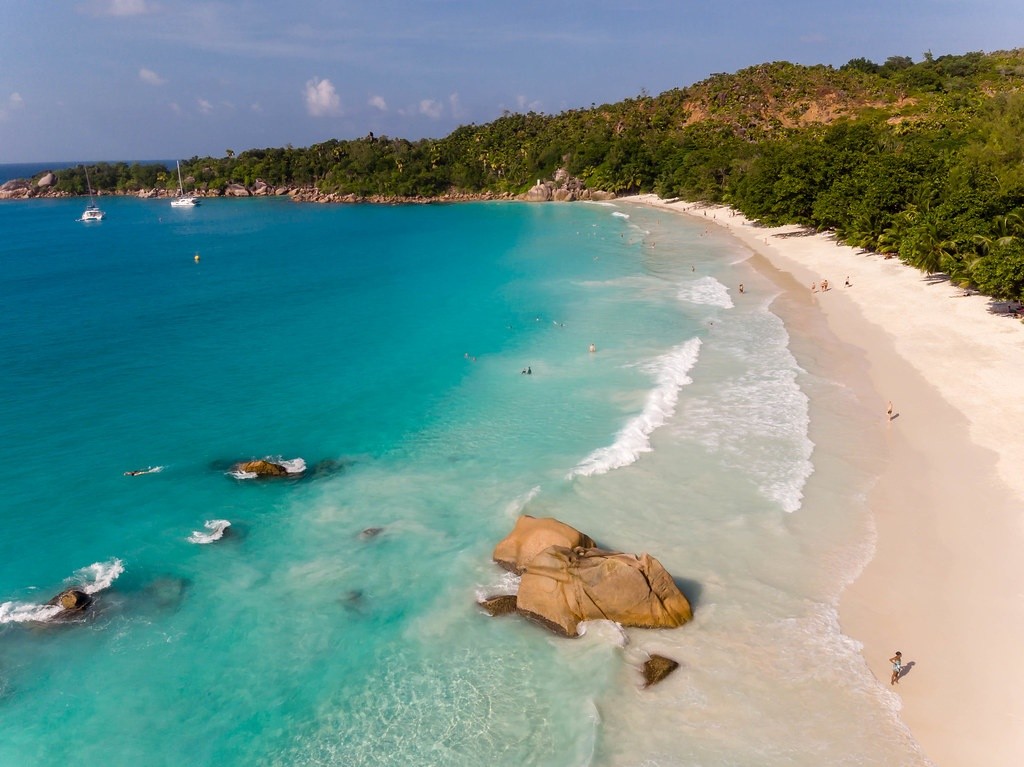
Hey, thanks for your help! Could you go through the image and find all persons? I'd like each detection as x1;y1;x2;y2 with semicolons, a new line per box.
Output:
890;652;902;685
691;266;694;271
522;367;531;374
845;276;850;287
573;196;745;246
739;284;743;292
887;400;893;422
765;238;767;246
590;344;595;352
812;280;828;291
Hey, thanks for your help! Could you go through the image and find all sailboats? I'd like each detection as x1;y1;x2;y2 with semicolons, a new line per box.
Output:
168;158;199;206
77;164;107;221
